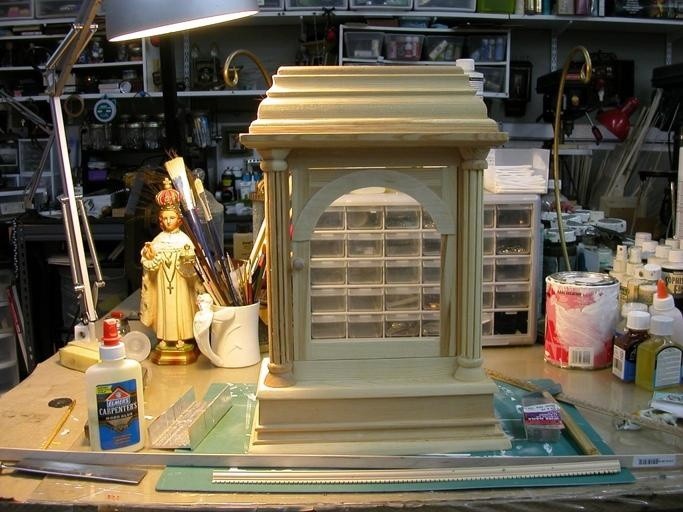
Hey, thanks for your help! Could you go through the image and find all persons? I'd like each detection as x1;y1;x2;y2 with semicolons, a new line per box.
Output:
138;177;206;350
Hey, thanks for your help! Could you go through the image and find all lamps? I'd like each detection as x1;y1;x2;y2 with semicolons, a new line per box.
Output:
43;0;264;369
593;96;644;143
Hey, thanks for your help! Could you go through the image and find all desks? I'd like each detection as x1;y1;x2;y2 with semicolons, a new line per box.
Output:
1;269;683;512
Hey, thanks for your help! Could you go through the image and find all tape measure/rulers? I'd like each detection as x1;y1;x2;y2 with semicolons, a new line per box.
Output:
484;367;683;440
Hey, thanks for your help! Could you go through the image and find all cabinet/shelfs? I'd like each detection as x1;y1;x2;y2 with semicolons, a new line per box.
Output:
0;0;683;103
312;189;540;349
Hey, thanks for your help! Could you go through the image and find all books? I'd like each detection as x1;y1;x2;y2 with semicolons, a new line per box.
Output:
525;0;683;20
0;25;121;95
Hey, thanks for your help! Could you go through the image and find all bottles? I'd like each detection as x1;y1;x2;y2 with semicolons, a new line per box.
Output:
636;316;682;390
615;303;648;338
612;311;651;382
649;280;683;346
611;232;683;301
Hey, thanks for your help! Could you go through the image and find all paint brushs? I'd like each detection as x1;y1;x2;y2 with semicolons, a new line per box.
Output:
41;399;76;451
164;155;293;307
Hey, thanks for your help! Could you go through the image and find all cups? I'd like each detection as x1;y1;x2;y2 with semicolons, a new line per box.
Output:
191;295;260;369
